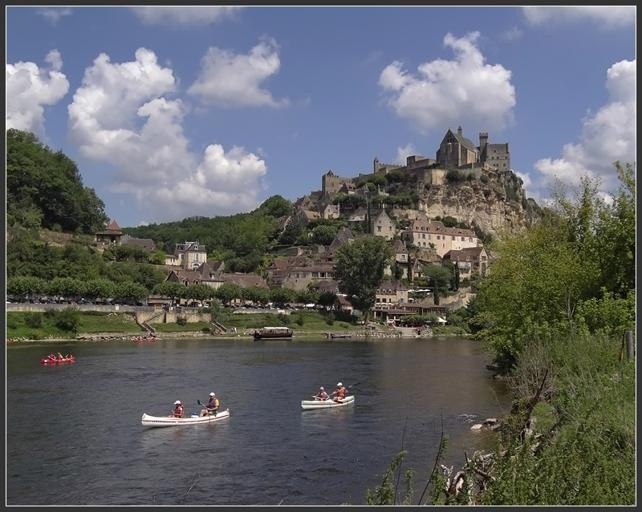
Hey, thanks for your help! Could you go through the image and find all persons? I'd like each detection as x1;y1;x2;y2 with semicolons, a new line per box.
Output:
333;382;347;401
48;351;55;360
200;392;219;417
57;351;63;359
313;386;329;400
169;400;183;418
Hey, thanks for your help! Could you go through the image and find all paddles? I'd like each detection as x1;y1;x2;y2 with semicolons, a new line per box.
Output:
197;399;208;408
330;382;360;397
313;396;344;404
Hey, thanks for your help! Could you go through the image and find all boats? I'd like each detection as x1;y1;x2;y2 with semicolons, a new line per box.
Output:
141;408;230;428
131;336;157;343
300;395;355;411
253;326;294;340
40;356;75;364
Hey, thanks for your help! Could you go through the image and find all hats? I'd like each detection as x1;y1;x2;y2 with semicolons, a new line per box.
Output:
337;382;343;386
209;392;216;397
319;387;325;390
173;400;181;405
